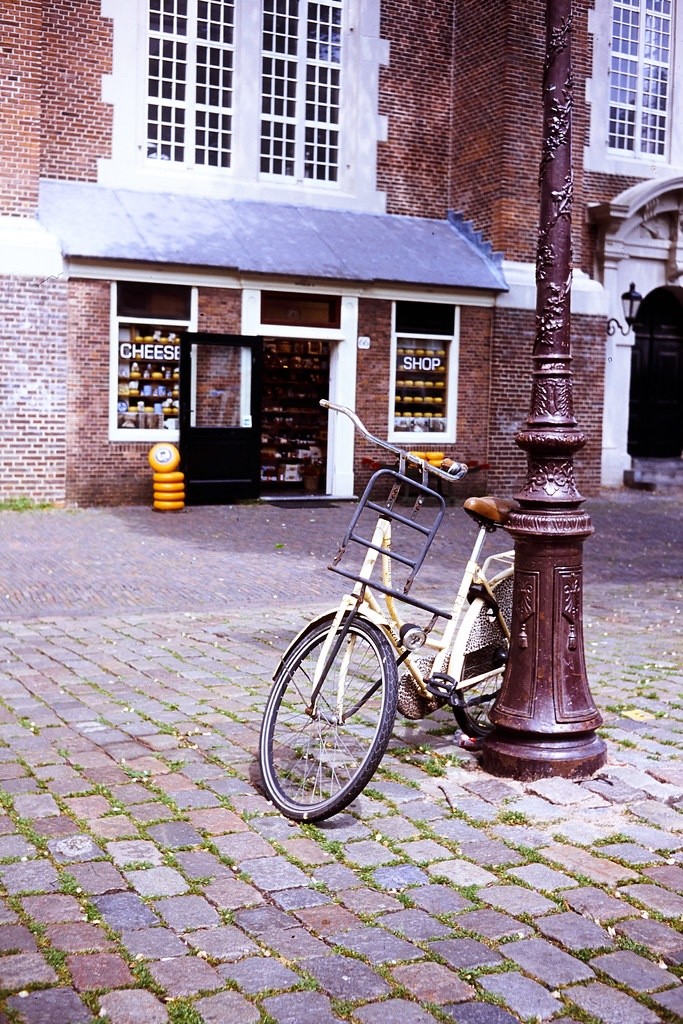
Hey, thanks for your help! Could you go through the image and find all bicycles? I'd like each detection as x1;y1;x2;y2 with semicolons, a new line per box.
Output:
259;399;533;827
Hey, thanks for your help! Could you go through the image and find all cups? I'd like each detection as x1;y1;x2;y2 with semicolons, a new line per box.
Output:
143;385;152;396
137;401;145;413
154;403;162;414
157;386;166;396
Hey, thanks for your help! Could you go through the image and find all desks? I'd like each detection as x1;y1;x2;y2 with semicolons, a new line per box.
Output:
362;458;489;504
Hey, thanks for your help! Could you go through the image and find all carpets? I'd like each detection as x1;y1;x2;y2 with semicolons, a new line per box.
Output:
268;499;338;508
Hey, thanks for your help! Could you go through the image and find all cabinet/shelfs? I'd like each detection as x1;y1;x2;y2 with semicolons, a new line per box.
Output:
394;338;450;418
261;351;330;490
117;322;187;416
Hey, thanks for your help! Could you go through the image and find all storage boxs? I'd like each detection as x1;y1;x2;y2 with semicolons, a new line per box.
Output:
279;464;302;481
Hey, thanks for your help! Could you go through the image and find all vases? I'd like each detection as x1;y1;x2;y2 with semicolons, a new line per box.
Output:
303;475;319;490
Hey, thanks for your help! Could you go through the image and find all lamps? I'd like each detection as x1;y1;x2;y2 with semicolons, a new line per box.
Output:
606;282;642;335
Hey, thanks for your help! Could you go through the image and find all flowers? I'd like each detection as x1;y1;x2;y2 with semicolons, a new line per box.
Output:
298;456;324;479
